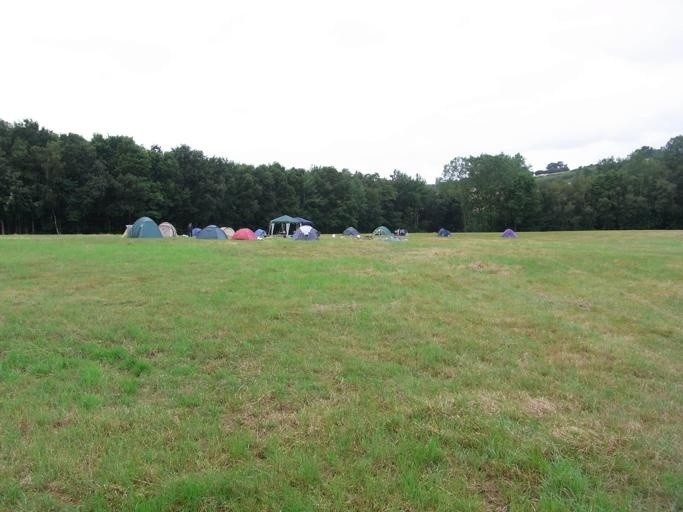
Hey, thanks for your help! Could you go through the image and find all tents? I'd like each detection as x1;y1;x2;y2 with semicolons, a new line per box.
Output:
502;229;516;237
191;227;201;235
372;226;391;235
254;229;266;236
343;226;358;235
230;228;256;240
125;216;163;238
221;226;234;236
292;225;317;239
159;222;176;236
195;224;227;239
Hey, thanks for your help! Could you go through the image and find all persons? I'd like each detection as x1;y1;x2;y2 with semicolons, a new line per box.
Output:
317;230;320;236
186;222;192;236
